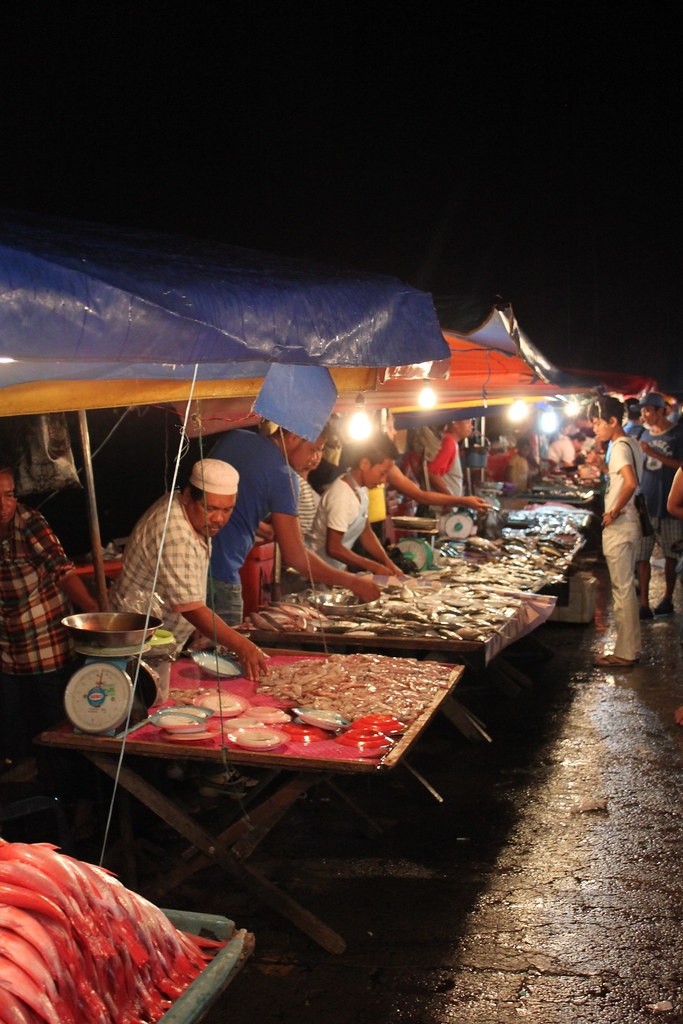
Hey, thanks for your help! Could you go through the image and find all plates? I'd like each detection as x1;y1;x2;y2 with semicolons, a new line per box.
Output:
191;650;243;679
147;689;409;752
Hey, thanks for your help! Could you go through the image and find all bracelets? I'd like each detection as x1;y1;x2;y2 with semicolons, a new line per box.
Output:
610;511;620;519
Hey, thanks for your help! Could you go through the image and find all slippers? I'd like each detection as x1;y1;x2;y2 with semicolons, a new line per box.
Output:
592;654;640;668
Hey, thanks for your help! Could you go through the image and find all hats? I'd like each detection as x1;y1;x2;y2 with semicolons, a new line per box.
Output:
637;393;668;408
189;458;240;496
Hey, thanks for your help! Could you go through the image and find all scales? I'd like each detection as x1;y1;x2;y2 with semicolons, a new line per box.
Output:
60;613;164;737
444;507;474;542
478;482;503;517
392;516;440;570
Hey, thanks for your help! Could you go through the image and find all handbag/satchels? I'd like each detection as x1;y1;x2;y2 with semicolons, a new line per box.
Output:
634;493;654;537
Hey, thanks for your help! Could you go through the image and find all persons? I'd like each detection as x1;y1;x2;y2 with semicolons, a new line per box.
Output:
294;435;403;579
415;416;472;540
507;436;531;493
107;458;271;681
546;413;588;467
366;463;492;548
0;463;101;797
588;392;683;668
202;422;382;627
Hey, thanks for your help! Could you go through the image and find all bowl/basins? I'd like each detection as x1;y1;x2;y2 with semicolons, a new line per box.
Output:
477;482;504;490
307;594;379;616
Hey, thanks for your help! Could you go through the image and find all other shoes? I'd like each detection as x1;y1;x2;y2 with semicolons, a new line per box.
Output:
654;599;674;614
639;606;653;620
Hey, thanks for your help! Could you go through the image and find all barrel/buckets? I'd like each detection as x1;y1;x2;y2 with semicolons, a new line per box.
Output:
464;447;488;467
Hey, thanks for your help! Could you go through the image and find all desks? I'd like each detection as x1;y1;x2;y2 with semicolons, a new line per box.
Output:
40;485;595;1024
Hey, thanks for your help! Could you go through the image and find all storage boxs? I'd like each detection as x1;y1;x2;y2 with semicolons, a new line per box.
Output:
238;538;277;614
549;571;596;624
